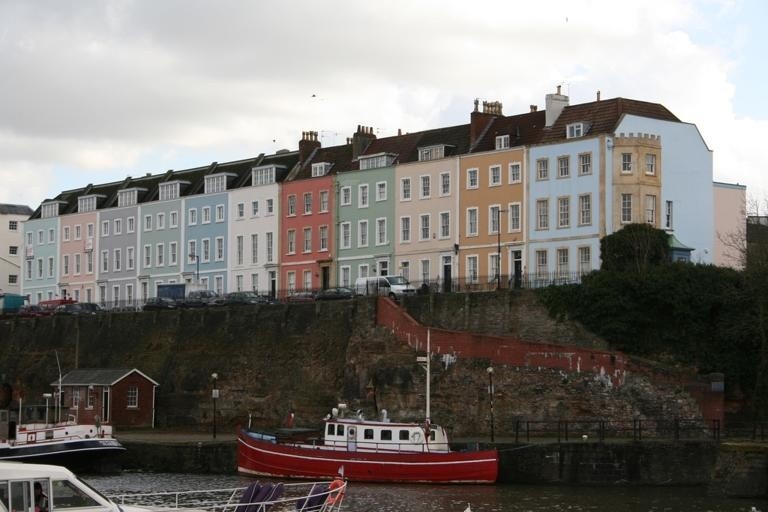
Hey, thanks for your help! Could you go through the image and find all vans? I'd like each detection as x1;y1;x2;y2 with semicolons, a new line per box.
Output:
352;273;418;297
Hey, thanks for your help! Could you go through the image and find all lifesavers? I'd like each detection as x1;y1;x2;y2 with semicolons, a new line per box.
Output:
327;479;344;505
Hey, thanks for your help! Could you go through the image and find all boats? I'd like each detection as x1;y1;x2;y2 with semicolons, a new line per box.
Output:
0;357;127;471
0;460;349;511
238;329;501;485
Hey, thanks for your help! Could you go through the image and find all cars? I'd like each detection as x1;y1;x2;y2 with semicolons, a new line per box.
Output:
141;296;178;311
22;299;103;314
175;285;351;309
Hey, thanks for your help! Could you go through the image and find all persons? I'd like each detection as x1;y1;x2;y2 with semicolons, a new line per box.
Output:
34;482;48;512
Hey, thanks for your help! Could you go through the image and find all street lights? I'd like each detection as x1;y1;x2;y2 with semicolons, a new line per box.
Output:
486;364;495;442
188;254;200;294
207;370;222;439
497;209;511;288
